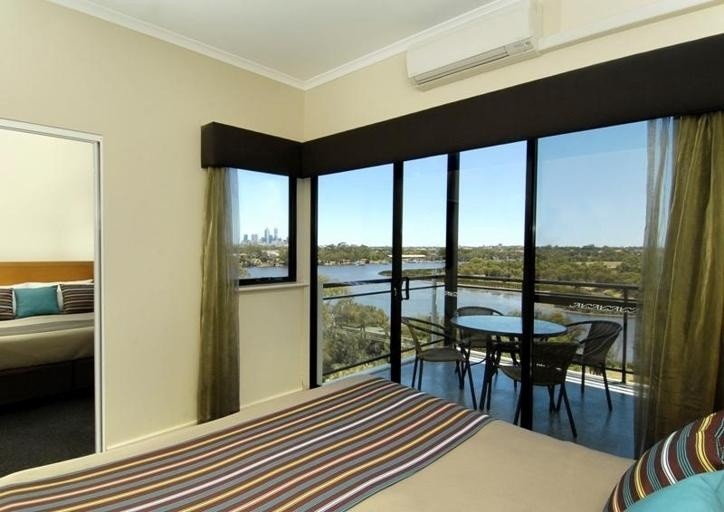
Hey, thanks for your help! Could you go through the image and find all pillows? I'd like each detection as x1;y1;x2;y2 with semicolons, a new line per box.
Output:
60;282;93;314
604;411;723;512
13;285;59;317
626;470;724;512
0;288;14;320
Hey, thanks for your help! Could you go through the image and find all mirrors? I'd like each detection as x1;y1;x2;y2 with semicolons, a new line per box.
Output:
0;118;105;479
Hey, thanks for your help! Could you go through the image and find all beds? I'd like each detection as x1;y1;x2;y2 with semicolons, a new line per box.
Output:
1;375;641;511
1;262;94;423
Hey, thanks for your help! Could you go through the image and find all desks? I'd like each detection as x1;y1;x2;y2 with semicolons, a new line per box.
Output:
451;314;568;409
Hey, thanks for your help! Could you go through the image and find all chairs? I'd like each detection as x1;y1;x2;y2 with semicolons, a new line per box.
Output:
453;306;514;390
486;334;578;438
552;321;622;411
400;315;477;410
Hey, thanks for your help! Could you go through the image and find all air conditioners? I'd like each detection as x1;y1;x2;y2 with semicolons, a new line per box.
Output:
405;1;541;94
